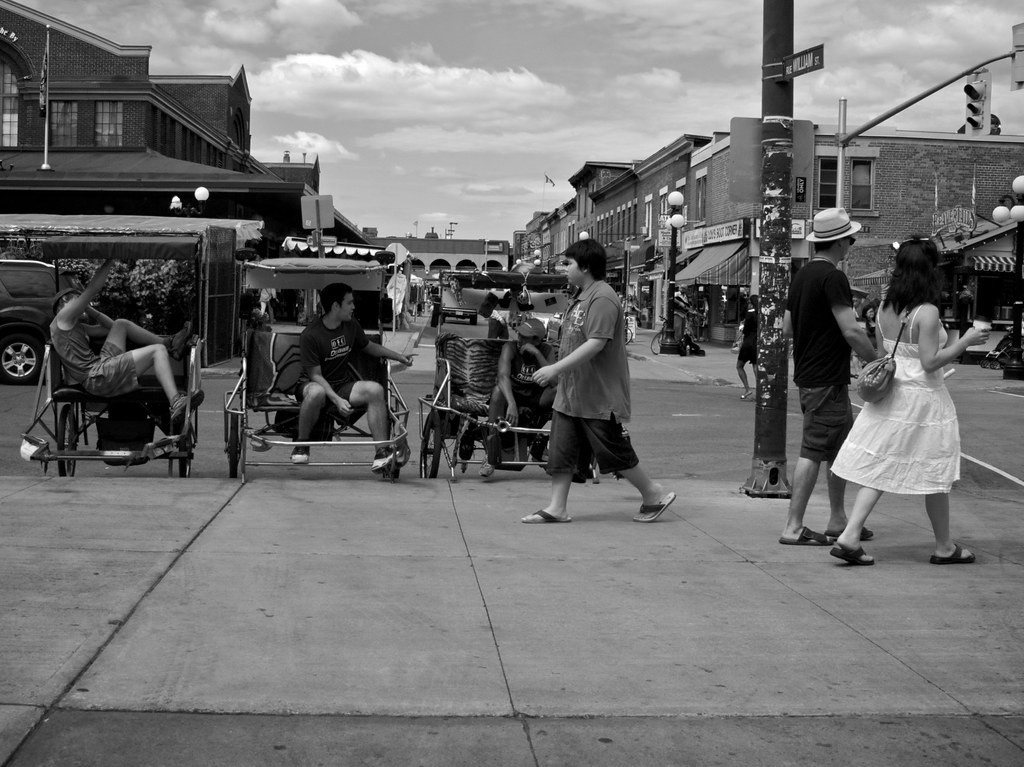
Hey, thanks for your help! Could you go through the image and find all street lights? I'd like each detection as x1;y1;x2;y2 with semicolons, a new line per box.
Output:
660;190;684;355
992;174;1024;382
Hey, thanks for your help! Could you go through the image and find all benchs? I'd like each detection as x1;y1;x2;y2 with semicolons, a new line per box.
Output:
251;324;389;411
444;339;559;419
52;325;191;405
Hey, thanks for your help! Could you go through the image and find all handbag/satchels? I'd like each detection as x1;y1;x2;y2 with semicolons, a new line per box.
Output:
857;357;896;402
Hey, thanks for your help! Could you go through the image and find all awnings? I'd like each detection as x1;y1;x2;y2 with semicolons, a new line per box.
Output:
630;242;752;286
970;256;1017;272
853;259;953;286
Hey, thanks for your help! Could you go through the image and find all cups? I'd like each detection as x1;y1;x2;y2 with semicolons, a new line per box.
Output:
973;316;992;332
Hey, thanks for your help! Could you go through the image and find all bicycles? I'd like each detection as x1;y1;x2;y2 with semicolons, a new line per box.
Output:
650;315;667;356
624;311;632;344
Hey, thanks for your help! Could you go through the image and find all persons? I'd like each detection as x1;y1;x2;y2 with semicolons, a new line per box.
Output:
522;239;676;524
259;288;279;324
831;236;992;565
291;283;418;474
488;287;509;340
736;295;758;398
49;259;205;422
780;207;878;545
850;305;880;378
674;283;689;341
621;295;639;318
477;319;558;477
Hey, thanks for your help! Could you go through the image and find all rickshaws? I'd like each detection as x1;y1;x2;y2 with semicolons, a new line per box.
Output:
224;258;410;482
19;234;206;478
417;267;600;486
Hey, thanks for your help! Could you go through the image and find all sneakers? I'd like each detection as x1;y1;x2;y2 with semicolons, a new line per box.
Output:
166;321;192;361
169;389;205;421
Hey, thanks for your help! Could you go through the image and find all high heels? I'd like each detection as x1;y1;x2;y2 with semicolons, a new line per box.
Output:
740;392;752;401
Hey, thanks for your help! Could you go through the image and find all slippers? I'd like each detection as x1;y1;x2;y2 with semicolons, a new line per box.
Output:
522;510;572;524
829;545;875;566
633;492;676;522
778;527;835;546
930;544;976;565
824;527;873;540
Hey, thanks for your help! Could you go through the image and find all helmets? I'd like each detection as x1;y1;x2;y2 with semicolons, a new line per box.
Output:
517;292;528;303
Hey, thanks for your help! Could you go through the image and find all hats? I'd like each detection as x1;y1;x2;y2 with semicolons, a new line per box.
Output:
53;288;83;314
806;207;861;242
515;319;545;337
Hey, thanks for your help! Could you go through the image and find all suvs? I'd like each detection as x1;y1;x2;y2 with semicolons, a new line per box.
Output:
0;259;98;386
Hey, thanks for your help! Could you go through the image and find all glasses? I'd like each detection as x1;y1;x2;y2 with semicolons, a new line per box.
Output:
841;237;857;245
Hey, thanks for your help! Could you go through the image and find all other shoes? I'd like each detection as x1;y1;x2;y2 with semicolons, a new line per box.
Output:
371;446;400;474
478;454;495;477
291;447;310;464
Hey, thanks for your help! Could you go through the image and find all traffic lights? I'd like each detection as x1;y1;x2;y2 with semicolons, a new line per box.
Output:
963;71;991;134
